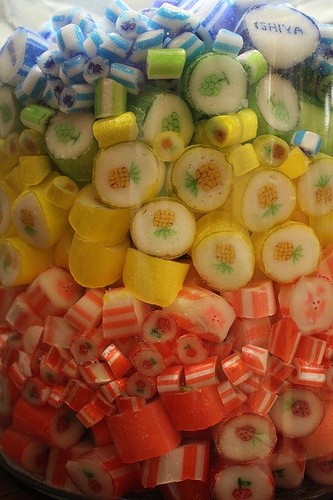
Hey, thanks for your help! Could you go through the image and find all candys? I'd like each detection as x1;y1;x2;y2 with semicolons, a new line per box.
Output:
1;1;333;500
